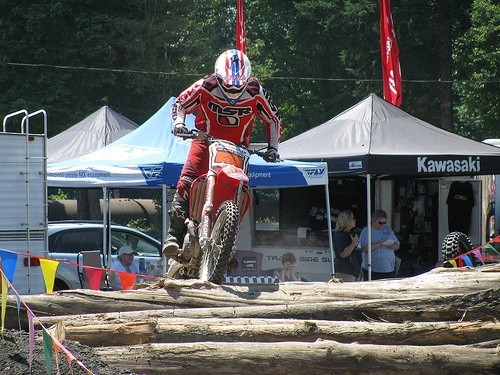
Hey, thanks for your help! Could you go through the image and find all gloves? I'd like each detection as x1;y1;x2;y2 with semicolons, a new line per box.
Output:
265;149;279;162
173;125;188;141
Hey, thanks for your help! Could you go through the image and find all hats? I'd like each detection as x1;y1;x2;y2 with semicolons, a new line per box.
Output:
119;246;137;256
282;253;296;264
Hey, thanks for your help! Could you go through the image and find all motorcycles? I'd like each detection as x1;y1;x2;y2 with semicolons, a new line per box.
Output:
168;129;285;286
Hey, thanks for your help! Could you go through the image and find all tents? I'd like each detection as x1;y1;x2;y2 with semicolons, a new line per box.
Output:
47;105;140;164
259;93;500;281
44;96;336;279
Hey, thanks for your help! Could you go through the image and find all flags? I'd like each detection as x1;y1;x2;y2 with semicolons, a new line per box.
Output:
379;0;402;106
236;0;246;54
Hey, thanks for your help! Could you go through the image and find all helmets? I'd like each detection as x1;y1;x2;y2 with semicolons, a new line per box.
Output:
215;49;252;105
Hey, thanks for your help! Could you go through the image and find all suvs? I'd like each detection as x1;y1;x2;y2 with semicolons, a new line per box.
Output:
48;220;164;291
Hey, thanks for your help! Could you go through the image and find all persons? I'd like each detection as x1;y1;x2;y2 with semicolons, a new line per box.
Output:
162;50;280;257
109;246;139;291
359;209;400;281
280;252;311;282
331;211;362;282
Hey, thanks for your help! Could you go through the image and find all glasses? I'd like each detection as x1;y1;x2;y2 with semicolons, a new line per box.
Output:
378;220;386;224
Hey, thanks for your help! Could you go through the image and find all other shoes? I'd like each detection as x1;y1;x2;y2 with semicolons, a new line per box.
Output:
162;235;179;257
